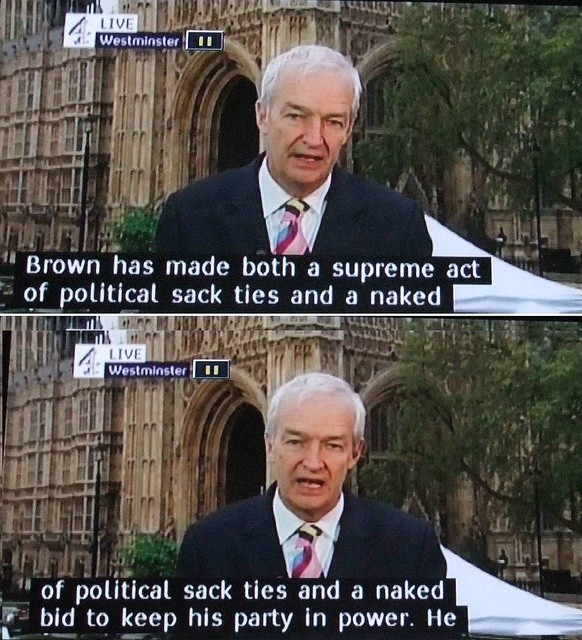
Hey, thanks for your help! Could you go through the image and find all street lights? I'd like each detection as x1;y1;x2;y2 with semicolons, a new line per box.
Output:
529;461;546;599
91;433;105;579
529;138;543;277
77;111;97;253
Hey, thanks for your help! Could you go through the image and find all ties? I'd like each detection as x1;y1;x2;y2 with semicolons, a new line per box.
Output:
275;198;314;255
288;523;324;580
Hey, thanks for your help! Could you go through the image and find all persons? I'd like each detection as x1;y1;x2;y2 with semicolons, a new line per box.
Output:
498;550;507;580
155;45;432;257
498;227;506;259
176;373;446;581
